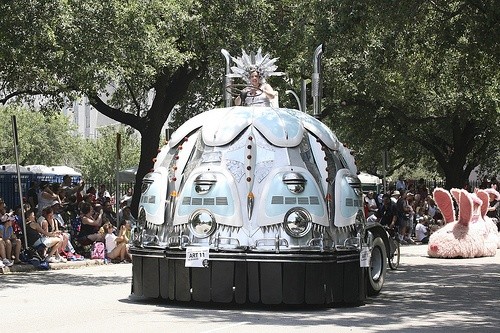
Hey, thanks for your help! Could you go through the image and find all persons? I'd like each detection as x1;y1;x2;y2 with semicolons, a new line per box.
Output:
365;175;445;244
235;65;275;106
479;175;500;231
0;174;133;267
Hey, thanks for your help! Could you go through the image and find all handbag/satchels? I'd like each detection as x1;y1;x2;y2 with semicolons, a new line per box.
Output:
91;242;104;259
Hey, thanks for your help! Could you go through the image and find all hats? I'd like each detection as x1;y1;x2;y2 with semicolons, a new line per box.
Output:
407;192;414;196
391;191;401;196
484;188;500;201
51;200;60;207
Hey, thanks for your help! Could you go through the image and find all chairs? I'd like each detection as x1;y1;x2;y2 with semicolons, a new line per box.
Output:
15;216;47;260
71;216;93;256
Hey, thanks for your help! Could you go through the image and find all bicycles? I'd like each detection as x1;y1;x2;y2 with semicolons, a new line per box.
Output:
380;225;401;269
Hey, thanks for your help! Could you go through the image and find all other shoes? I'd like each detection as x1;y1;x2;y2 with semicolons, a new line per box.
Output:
0;260;6;268
14;260;26;265
46;256;60;263
120;259;128;264
3;258;13;267
56;256;67;262
